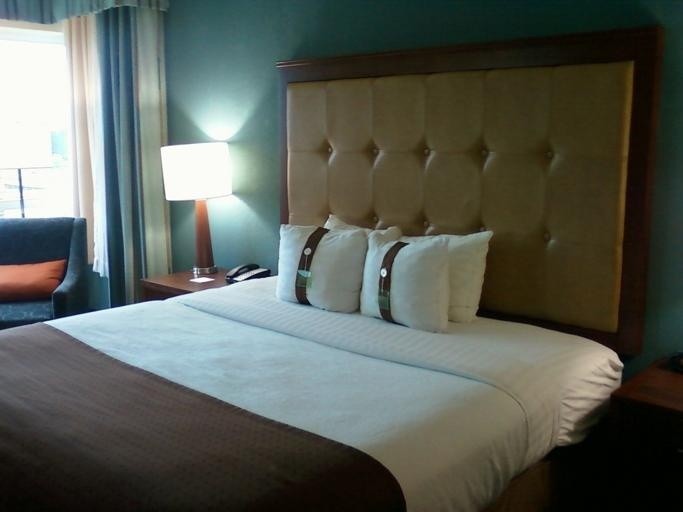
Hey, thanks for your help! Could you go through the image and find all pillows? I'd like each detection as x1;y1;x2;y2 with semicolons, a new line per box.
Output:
385;226;495;324
275;222;367;313
359;230;457;335
321;213;400;242
0;258;66;303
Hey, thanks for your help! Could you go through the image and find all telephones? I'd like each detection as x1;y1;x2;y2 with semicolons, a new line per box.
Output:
225;264;271;283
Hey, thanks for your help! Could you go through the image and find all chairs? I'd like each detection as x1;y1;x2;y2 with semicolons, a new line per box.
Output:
0;216;91;329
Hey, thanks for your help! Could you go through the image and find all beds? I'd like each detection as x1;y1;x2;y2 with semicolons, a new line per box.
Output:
0;273;667;512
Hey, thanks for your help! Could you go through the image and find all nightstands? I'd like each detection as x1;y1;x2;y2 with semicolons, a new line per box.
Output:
135;269;230;300
609;345;682;512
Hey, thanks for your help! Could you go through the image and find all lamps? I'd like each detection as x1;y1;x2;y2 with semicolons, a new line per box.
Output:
159;141;234;275
